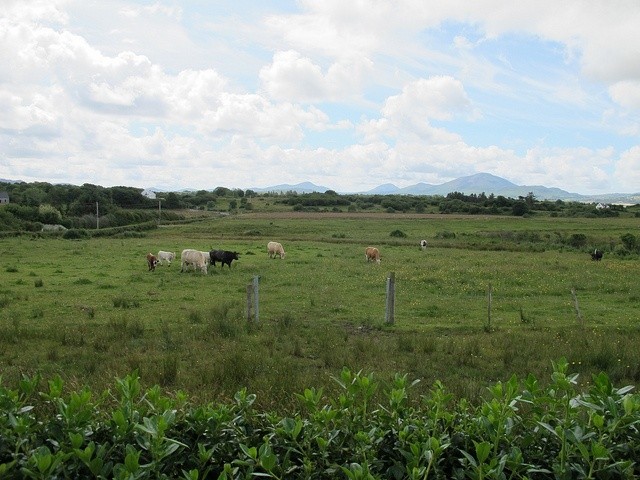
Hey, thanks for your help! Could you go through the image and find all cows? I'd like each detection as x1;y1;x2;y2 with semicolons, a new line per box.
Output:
365;247;383;264
210;249;239;268
158;251;176;268
591;249;602;261
267;242;285;260
179;249;207;275
147;253;159;272
203;252;210;265
420;240;427;250
